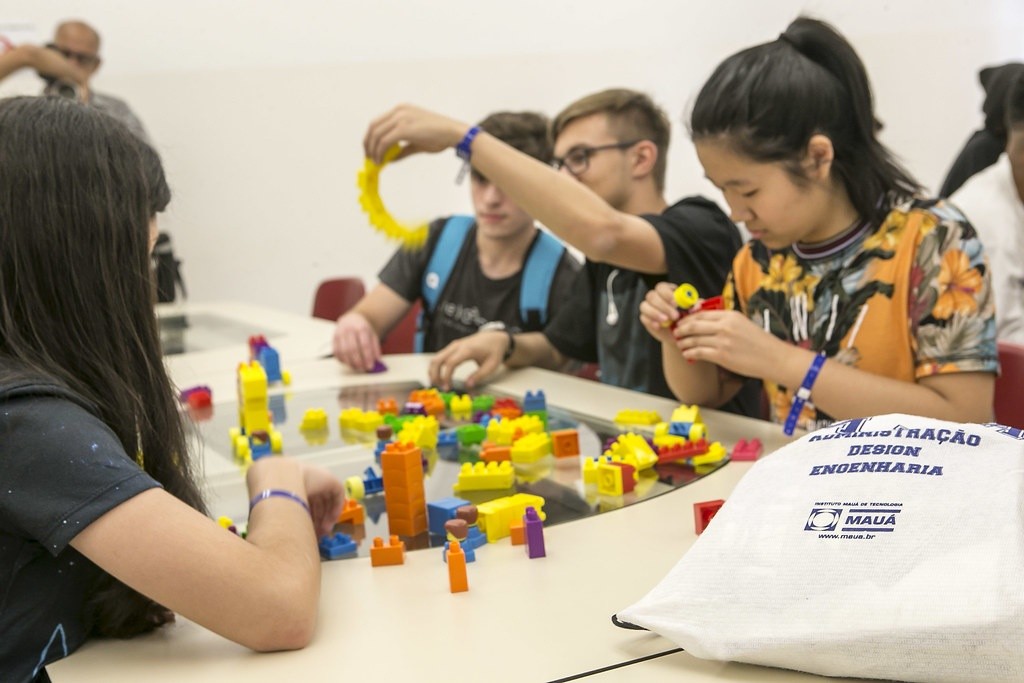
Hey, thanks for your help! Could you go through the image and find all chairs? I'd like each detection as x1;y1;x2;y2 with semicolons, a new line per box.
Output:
314;278;421;356
993;344;1024;429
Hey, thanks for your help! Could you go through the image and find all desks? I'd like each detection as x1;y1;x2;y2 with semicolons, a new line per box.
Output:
43;305;893;683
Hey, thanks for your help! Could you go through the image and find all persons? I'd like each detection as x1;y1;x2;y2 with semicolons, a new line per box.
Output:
639;13;1004;434
364;90;763;418
333;113;579;375
0;93;346;683
932;61;1023;347
0;18;141;140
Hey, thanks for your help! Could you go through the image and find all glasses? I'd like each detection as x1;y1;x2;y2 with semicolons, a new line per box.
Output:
546;140;639;177
50;45;96;68
469;163;492;189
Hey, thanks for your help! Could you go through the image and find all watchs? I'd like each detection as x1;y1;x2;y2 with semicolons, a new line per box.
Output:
474;320;515;368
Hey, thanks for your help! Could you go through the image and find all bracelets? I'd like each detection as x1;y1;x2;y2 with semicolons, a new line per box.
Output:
456;125;480;160
249;491;309;517
786;352;827;433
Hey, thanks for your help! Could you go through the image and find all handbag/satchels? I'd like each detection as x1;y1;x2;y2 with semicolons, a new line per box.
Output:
611;414;1024;683
